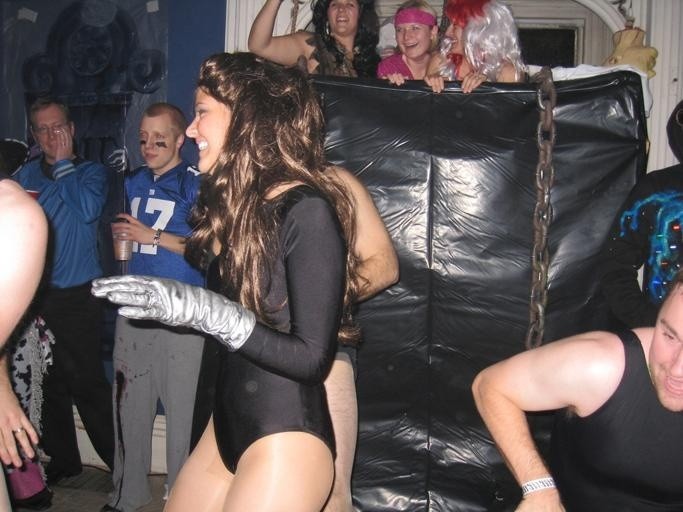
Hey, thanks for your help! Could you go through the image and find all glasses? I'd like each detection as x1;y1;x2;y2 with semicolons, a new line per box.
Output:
32;124;67;134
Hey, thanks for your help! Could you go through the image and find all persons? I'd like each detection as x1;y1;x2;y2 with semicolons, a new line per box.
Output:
247;0;380;80
374;0;441;86
599;97;683;325
469;265;683;512
1;92;116;511
423;0;526;96
92;50;399;511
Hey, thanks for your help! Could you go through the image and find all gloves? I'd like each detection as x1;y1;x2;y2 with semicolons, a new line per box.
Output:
90;273;256;352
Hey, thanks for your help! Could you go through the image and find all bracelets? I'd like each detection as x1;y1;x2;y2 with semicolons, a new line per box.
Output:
520;477;555;495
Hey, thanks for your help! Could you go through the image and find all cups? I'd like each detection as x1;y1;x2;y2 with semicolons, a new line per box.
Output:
110;223;134;261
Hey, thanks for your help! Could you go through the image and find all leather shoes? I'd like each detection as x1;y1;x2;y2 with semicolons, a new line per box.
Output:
45;465;82;484
